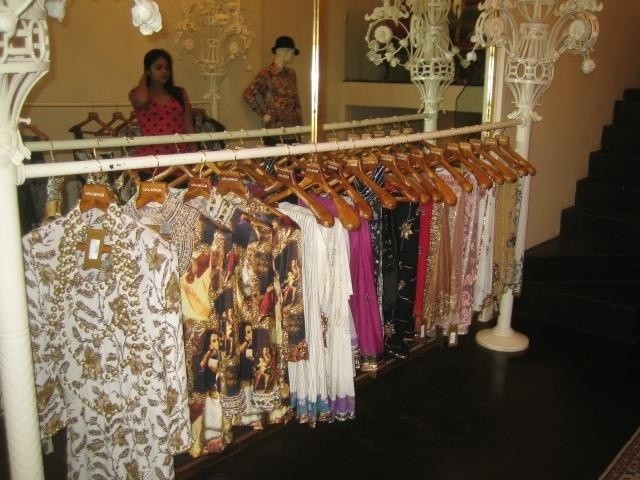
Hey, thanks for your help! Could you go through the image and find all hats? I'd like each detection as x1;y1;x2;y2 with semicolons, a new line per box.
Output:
272;36;299;55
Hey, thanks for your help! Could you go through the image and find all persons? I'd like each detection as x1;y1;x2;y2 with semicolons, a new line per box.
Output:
128;49;204;179
243;35;303;145
